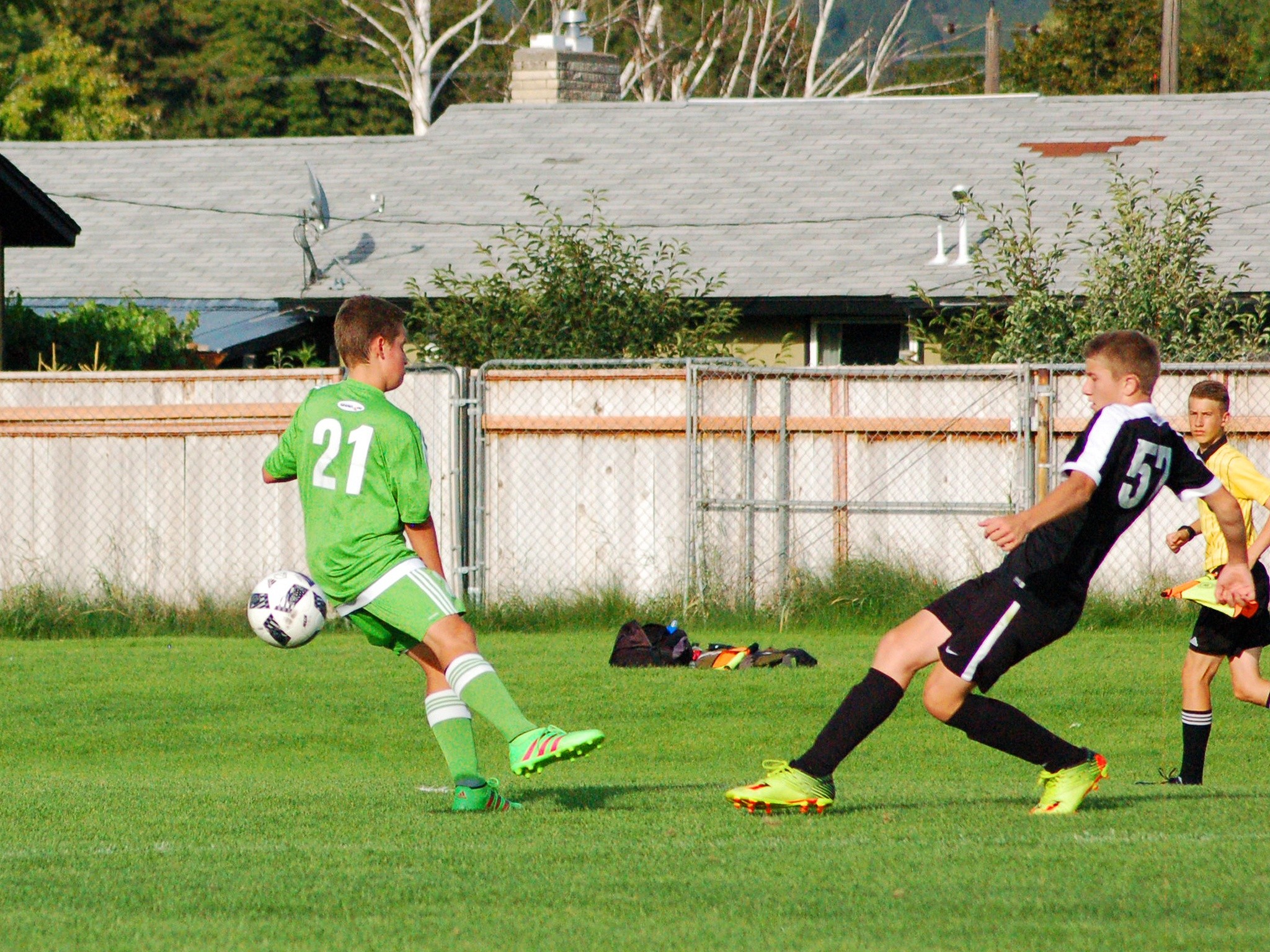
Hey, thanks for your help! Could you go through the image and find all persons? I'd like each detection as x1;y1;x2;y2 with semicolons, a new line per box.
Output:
725;331;1256;815
262;295;604;814
1136;380;1270;786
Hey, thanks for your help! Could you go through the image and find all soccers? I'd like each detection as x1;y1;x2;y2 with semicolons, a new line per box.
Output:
247;570;327;649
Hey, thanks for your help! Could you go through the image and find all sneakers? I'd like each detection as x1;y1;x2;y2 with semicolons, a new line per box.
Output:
1031;747;1110;816
726;766;835;813
509;725;606;780
454;783;526;814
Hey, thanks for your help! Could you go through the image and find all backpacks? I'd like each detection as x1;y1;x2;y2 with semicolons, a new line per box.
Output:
609;619;818;669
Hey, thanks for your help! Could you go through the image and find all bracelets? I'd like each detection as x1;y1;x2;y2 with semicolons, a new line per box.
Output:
1177;525;1195;541
